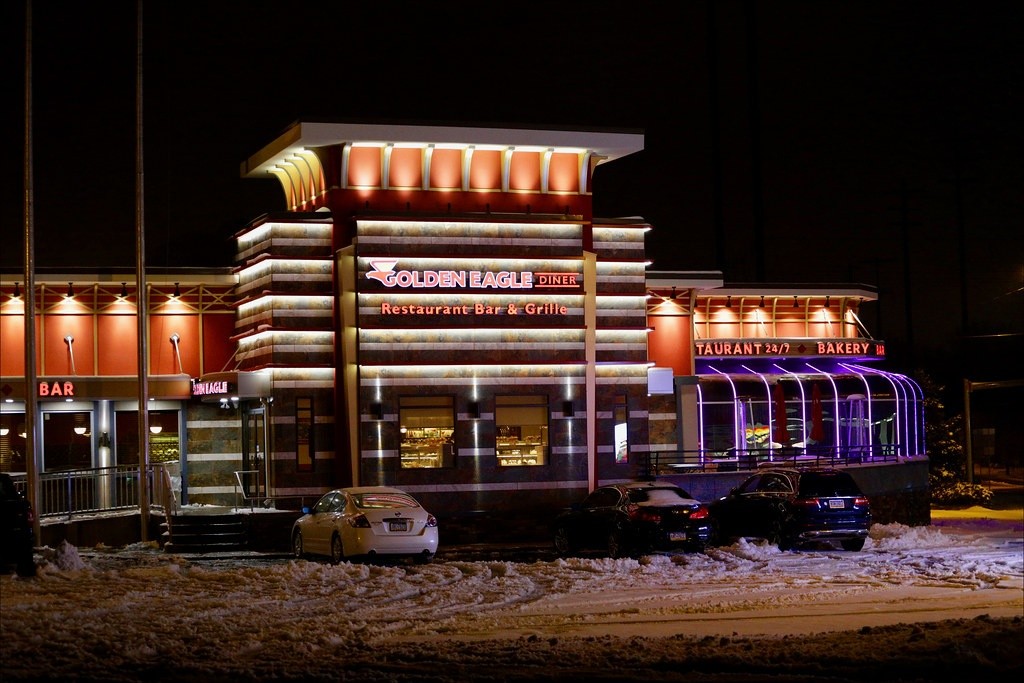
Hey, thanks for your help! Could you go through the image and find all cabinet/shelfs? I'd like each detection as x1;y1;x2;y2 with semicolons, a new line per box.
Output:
495;424;543;468
400;426;454;467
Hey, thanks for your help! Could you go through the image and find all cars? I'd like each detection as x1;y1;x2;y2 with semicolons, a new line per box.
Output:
291;486;439;565
552;481;713;558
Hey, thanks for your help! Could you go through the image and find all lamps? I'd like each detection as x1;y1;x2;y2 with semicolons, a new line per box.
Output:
667;286;865;309
13;280;181;298
0;413;163;439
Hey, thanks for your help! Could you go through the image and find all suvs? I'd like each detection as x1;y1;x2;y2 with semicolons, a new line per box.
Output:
713;467;870;551
0;474;33;577
